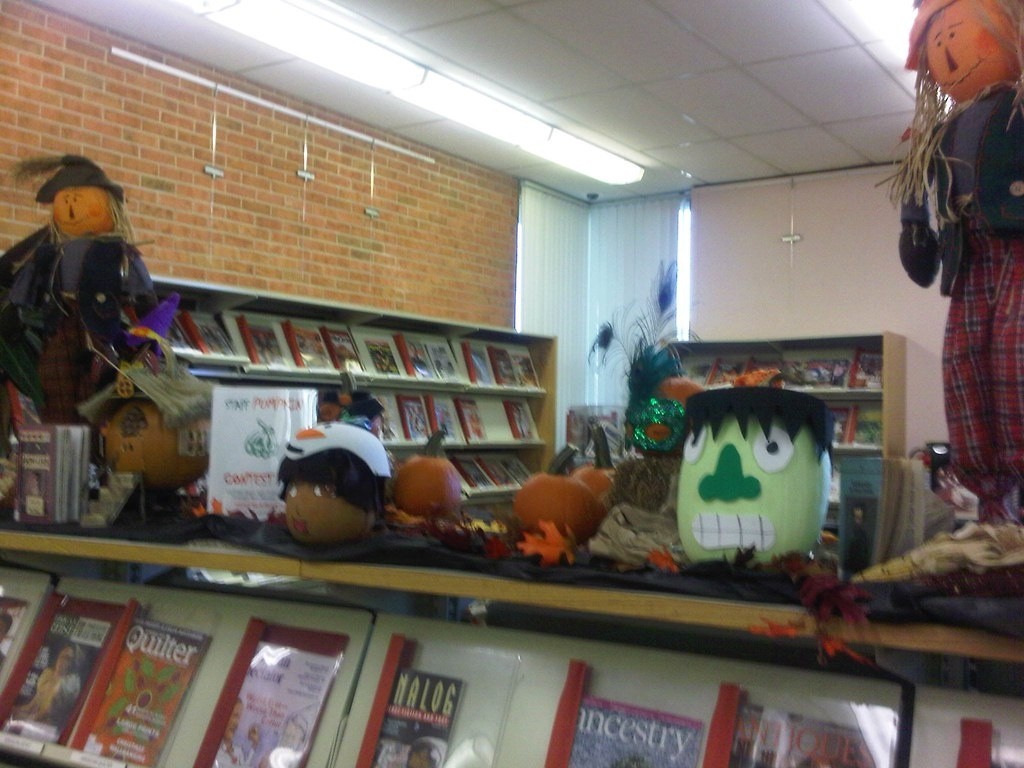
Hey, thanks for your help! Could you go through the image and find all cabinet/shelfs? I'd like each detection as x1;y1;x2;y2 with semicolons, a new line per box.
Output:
150;272;559;534
662;329;908;531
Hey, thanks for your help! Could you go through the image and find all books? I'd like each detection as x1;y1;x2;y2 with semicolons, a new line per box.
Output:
729;701;891;768
82;614;214;768
371;667;467;768
376;393;541;491
0;595;30;672
15;421;92;526
212;640;338;768
685;342;883;448
165;310;540;388
2;598;127;747
835;456;927;585
567;695;705;768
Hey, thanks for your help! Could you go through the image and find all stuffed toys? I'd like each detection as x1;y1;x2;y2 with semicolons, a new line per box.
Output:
1;152;166;468
873;0;1023;598
